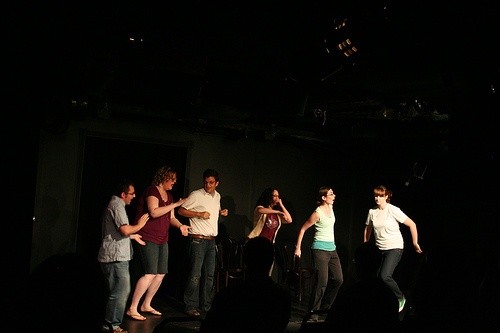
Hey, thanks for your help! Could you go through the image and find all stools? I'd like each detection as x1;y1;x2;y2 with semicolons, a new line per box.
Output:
295;267;316;302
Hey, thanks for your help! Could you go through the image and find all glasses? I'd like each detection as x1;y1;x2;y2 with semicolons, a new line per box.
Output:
127;191;136;195
272;195;281;198
205;180;215;184
169;178;176;182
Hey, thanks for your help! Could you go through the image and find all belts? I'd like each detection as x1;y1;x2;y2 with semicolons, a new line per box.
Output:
190;234;215;240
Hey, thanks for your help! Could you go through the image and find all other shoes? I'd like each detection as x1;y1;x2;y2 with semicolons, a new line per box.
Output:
104;325;128;333
398;297;406;312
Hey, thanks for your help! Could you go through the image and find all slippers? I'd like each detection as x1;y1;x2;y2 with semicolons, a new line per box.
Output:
141;309;163;315
125;313;146;321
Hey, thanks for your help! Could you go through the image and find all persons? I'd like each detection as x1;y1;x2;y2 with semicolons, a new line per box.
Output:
363;186;422;313
201;236;292;333
178;168;228;317
293;186;343;321
98;179;151;333
126;167;192;321
248;187;292;278
